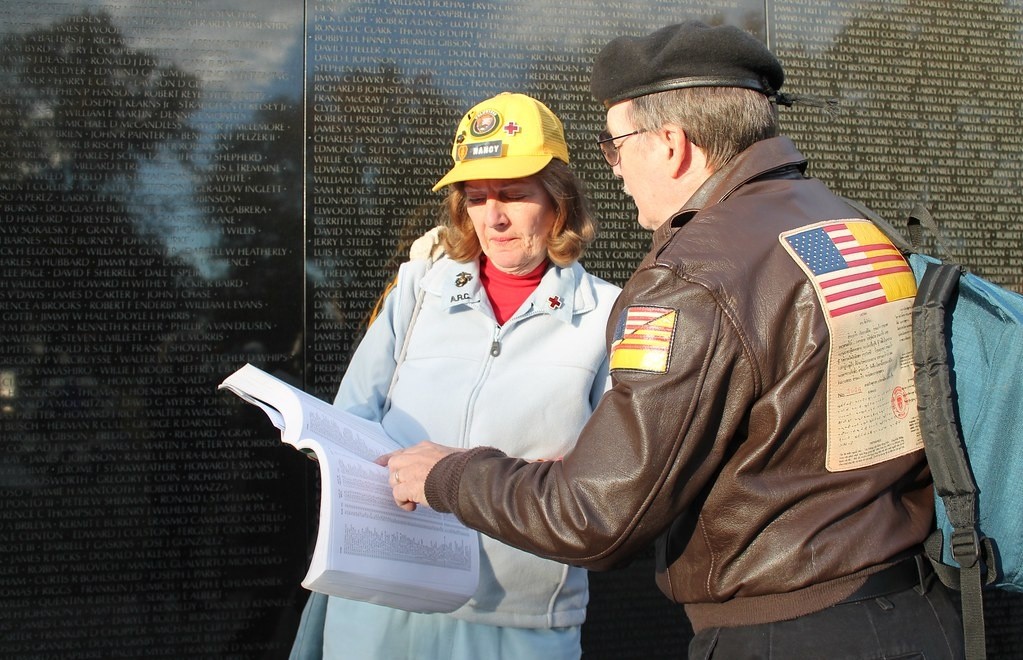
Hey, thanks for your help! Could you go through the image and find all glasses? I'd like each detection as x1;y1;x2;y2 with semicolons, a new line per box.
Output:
596;126;661;166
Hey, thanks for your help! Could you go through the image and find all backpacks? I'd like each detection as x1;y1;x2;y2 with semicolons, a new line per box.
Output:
846;193;1023;595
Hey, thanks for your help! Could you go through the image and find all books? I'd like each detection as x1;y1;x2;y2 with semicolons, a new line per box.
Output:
218;361;481;616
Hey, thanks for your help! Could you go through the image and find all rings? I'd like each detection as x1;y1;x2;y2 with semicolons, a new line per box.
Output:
395;472;400;483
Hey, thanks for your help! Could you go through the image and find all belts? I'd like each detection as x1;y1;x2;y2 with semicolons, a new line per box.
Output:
834;553;934;606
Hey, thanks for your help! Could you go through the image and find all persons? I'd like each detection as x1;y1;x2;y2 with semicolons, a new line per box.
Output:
367;20;964;660
289;92;623;660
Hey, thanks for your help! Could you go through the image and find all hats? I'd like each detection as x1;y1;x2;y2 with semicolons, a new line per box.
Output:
432;91;571;196
589;21;786;110
